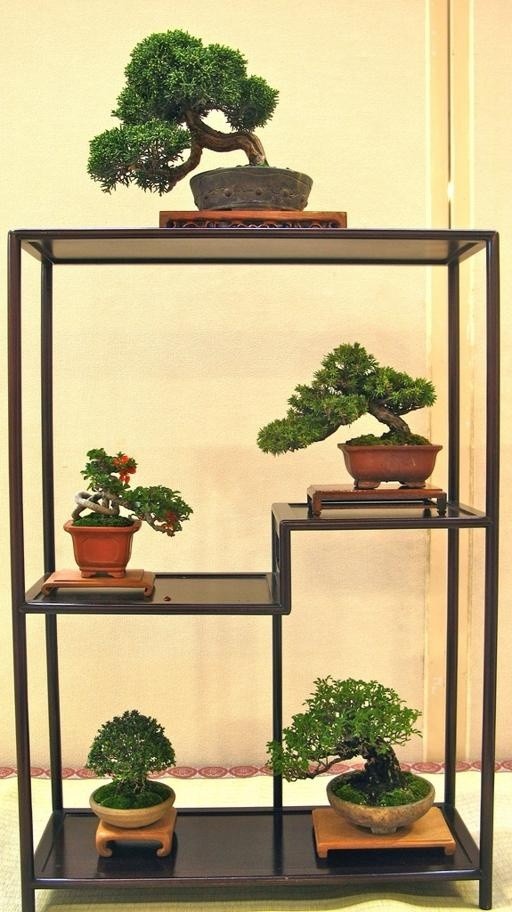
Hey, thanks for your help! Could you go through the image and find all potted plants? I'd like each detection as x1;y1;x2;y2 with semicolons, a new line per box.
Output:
63;447;195;579
85;27;314;211
84;710;178;859
255;340;443;489
264;673;437;836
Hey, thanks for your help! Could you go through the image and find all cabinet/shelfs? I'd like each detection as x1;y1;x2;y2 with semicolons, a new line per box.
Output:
8;228;501;905
